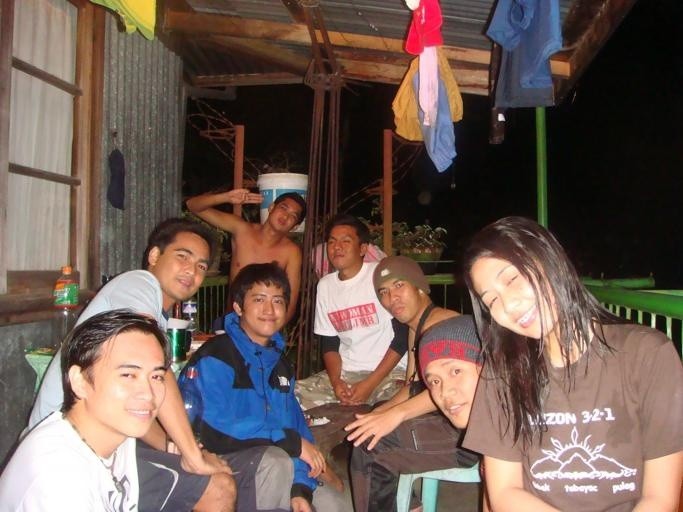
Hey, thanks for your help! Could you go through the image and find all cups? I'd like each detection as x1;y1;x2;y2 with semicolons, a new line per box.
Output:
166;328;191;363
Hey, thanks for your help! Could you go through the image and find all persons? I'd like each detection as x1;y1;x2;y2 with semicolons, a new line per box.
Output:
185;188;306;334
344;256;480;512
19;218;238;512
294;215;418;410
419;314;489;512
176;263;327;511
462;214;683;512
0;309;170;512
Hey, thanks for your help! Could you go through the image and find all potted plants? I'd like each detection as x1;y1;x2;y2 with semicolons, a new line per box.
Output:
397;225;446;274
180;210;232;278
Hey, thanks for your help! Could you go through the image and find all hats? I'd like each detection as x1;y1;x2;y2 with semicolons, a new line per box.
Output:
419;315;484;391
373;256;431;298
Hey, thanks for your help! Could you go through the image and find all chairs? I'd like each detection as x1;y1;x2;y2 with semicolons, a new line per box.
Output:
396;461;484;512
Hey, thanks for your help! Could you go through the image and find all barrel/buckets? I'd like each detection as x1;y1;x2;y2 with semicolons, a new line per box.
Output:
256;172;308;232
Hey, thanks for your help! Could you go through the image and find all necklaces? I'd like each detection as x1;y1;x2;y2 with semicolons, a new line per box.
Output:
65;416;125;495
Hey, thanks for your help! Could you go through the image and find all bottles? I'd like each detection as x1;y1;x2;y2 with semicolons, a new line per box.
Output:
182;292;199;333
51;266;79;350
166;367;203;455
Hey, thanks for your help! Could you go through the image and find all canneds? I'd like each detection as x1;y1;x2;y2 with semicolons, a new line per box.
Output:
166;327;186;363
